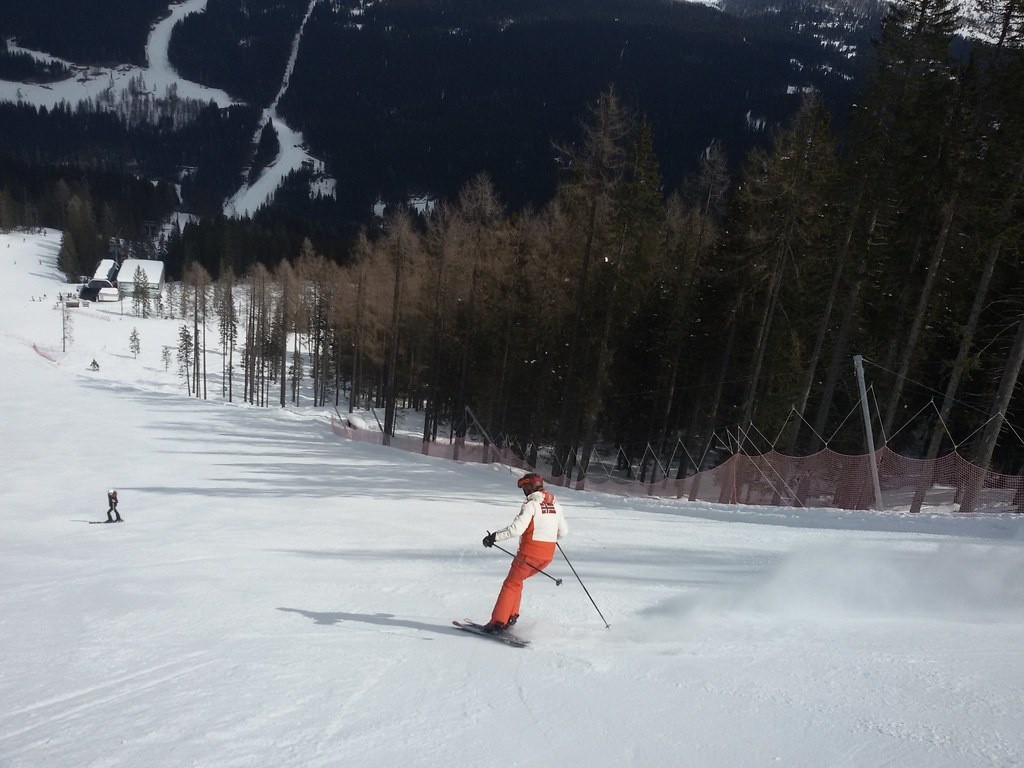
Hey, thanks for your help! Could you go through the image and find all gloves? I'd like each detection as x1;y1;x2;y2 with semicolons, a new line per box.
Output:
483;532;496;548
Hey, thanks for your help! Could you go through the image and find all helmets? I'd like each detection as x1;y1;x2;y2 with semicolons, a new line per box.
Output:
521;473;543;496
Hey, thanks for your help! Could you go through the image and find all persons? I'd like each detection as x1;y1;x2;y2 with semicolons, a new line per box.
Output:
481;473;568;632
105;489;122;523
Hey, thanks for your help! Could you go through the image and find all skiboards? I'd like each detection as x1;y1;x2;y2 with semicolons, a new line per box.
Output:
452;617;531;646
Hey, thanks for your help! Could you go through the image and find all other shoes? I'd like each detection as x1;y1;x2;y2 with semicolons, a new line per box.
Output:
504;615;519;630
480;622;504;635
